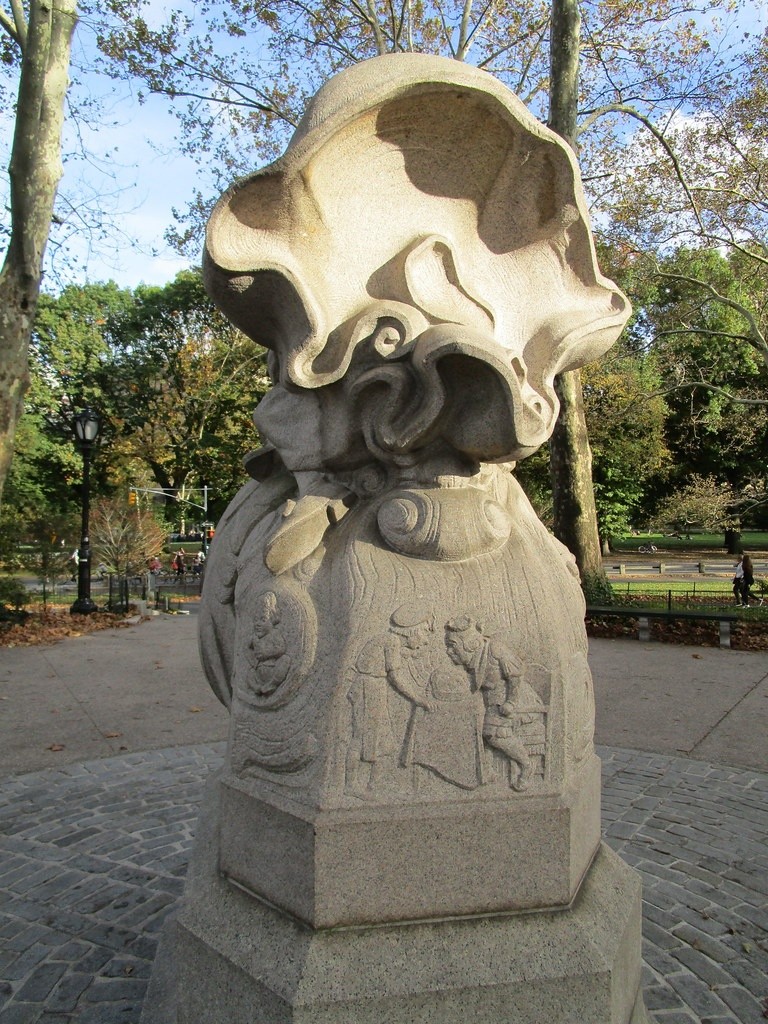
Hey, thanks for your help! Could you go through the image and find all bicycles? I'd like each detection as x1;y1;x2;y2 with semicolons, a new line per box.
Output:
638;540;658;555
88;571;113;583
56;565;83;585
147;559;205;586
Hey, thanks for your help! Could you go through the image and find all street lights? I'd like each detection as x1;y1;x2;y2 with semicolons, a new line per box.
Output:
69;405;103;618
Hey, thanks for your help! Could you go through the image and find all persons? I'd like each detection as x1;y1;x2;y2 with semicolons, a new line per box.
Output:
61;543;207;580
740;555;763;607
732;553;746;606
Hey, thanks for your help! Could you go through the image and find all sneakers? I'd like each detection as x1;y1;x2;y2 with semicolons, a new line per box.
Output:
742;604;750;608
757;598;763;607
735;603;743;607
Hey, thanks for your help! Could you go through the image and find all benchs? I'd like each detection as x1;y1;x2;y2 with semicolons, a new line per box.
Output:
585;604;739;648
